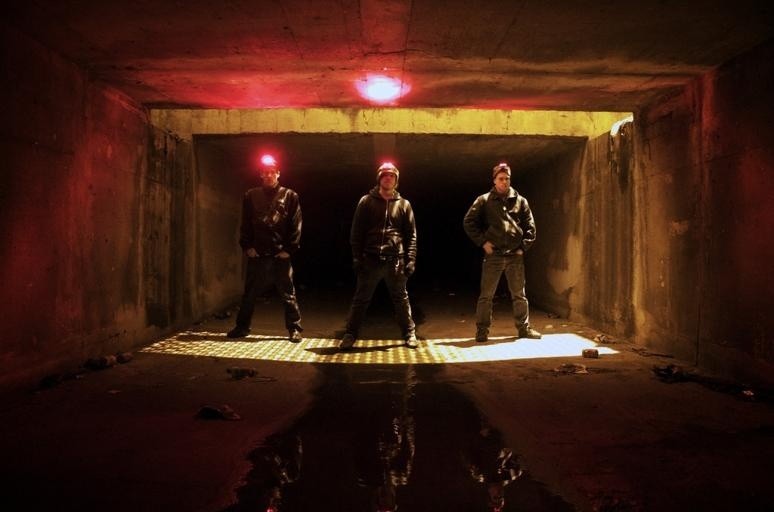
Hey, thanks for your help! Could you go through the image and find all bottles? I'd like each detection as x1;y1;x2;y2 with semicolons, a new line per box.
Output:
493;165;511;178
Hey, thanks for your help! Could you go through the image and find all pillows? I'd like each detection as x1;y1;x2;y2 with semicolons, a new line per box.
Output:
376;162;400;187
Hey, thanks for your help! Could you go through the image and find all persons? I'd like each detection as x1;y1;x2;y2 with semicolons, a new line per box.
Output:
463;162;542;342
226;157;304;343
340;162;420;348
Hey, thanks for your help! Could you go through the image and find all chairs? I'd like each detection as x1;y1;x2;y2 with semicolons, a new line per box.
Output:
227;327;249;337
476;329;488;342
406;337;418;348
289;328;302;343
340;334;354;348
519;329;541;339
